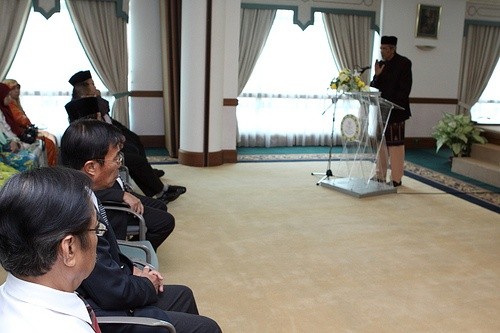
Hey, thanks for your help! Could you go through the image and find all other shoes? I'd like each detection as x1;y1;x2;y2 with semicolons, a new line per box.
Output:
387;180;401;187
155;186;186;205
155;170;164;177
370;178;387;183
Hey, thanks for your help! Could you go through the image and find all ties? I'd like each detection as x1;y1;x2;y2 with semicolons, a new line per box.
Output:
77;294;101;333
97;197;109;226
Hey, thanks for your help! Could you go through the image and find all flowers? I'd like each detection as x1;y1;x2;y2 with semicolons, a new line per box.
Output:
327;69;371;93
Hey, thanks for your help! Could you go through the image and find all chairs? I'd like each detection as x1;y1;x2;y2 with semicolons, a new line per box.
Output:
41;132;176;333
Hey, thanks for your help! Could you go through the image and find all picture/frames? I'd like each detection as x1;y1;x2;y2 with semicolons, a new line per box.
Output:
415;3;442;40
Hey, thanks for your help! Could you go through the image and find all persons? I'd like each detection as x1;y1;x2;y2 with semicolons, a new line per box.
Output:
60;120;223;333
0;167;107;333
65;97;175;252
0;82;48;191
370;35;413;186
69;70;187;203
3;79;57;147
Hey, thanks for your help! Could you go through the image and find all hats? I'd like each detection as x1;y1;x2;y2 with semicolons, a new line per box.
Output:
64;97;110;122
68;70;92;87
381;36;397;46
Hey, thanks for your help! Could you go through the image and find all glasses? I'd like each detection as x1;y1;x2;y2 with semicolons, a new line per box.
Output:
82;152;123;166
59;221;108;244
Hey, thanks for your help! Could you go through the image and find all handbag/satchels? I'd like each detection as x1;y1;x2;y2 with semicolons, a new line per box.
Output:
17;126;38;145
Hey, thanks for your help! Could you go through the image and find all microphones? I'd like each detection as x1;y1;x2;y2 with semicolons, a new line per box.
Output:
360;66;370;69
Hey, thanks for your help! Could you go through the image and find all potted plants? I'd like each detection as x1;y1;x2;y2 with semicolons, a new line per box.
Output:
431;111;488;162
